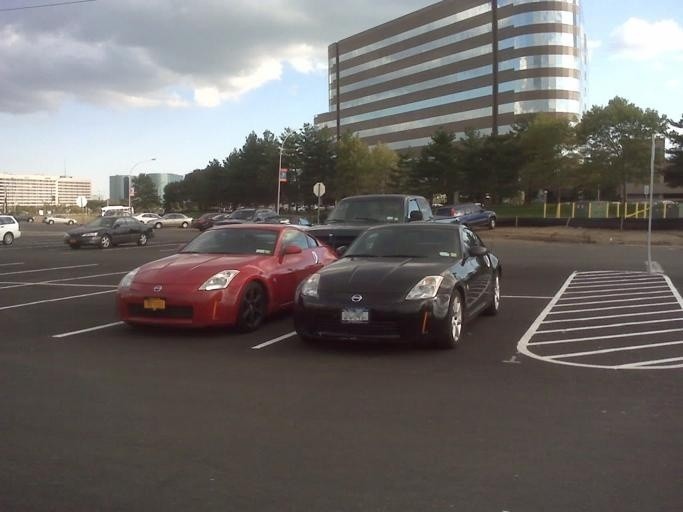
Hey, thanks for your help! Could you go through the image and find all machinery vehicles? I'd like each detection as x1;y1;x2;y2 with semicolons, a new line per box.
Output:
291;223;502;351
112;223;336;332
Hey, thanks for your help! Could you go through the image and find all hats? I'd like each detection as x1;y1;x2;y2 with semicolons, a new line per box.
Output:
62;215;154;249
433;203;497;230
190;212;231;231
131;213;161;224
246;213;311;228
43;214;77;225
147;213;192;228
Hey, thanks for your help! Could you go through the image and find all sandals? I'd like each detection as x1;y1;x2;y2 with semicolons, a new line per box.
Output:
99;205;134;216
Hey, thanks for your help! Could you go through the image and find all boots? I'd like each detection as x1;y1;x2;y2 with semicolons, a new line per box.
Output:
0;214;20;246
304;193;434;260
212;208;277;229
10;211;35;223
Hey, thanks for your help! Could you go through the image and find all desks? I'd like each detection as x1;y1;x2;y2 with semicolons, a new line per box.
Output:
552;168;560;199
275;124;312;214
128;158;156;207
647;133;660;274
